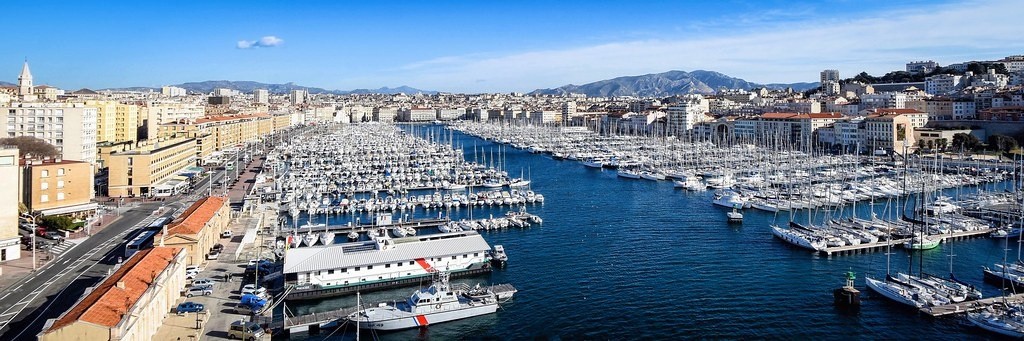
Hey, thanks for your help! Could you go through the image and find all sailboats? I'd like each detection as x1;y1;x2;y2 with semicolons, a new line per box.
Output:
293;210;335;247
348;209;416;242
446;120;838;180
966;301;1024;338
281;124;544;217
864;272;983;309
673;155;1023;253
438;211;543;233
982;218;1024;293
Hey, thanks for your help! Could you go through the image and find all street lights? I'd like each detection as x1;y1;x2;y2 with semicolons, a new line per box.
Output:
251;142;253;161
23;212;45;272
236;148;239;180
209;166;212;196
244;140;246;168
224;159;229;198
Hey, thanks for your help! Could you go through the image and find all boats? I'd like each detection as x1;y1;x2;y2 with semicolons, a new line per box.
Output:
347;271;500;331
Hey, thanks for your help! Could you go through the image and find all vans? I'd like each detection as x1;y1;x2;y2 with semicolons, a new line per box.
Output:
228;320;265;341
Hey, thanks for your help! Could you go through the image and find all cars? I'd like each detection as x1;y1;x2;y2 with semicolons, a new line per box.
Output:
19;222;61;250
186;266;200;279
207;244;224;260
175;302;204;314
233;283;268;316
227;161;236;170
185;278;215;298
223;230;232;238
